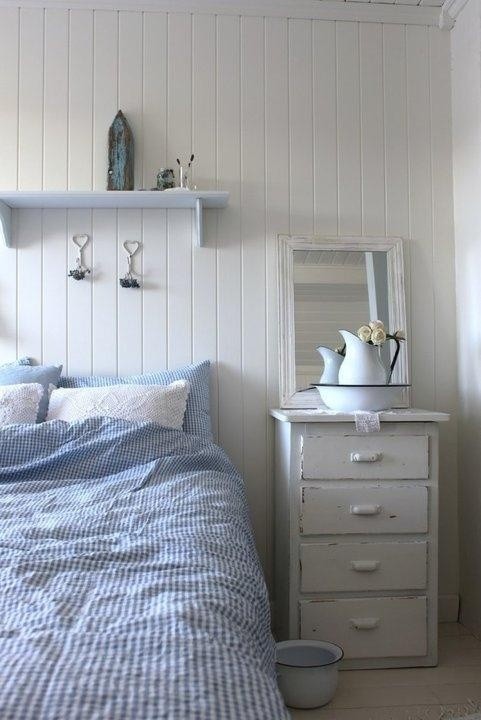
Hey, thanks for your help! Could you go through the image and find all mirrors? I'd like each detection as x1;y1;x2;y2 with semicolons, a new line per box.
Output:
273;230;409;410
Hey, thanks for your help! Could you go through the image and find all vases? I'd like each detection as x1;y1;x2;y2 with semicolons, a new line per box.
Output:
314;347;345;384
336;329;398;385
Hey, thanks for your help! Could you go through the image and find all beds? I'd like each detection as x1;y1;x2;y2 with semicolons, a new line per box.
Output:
1;356;290;719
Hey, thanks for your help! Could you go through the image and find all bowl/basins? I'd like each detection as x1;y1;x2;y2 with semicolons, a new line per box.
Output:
310;384;412;411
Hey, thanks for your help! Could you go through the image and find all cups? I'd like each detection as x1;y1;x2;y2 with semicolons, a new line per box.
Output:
179;166;196;192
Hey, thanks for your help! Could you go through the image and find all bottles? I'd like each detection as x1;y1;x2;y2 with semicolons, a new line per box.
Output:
157;168;175;191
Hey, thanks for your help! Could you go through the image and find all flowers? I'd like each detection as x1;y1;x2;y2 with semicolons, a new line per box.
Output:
359;317;388;345
335;327;408;355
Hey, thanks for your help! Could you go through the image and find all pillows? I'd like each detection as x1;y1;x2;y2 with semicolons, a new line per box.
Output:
2;360;215;440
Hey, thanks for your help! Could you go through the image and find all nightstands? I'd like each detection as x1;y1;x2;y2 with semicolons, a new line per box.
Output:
268;406;452;672
